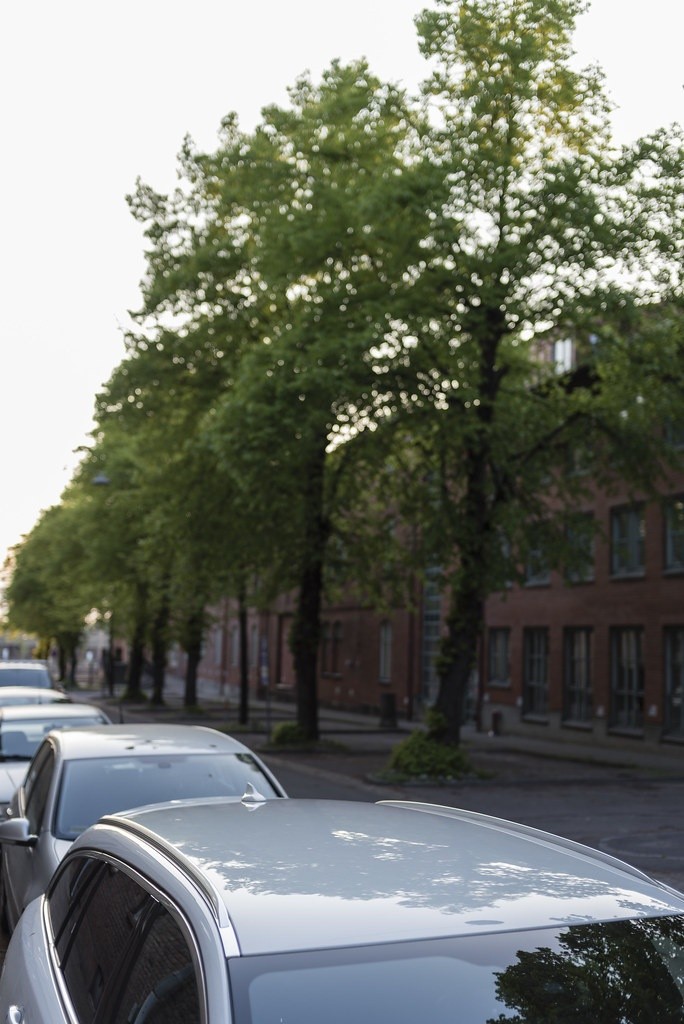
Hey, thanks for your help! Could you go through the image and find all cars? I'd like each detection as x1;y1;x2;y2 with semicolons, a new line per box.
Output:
1;656;120;809
0;723;297;953
0;795;684;1024
